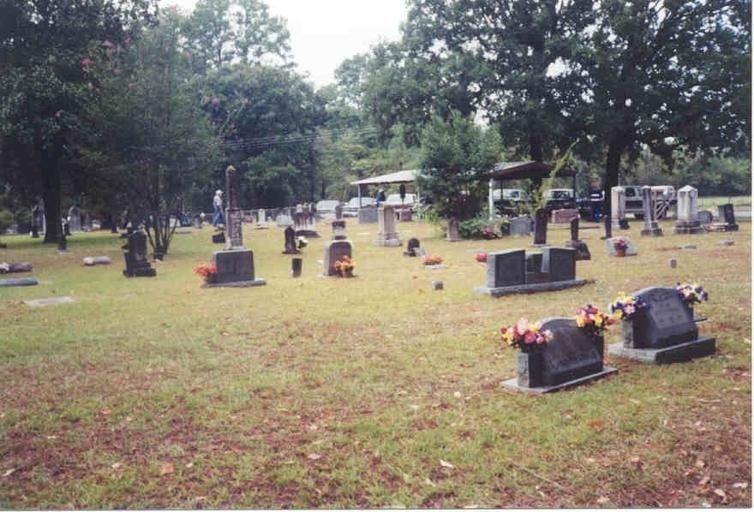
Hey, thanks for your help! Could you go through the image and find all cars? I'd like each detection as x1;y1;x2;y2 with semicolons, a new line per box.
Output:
317;188;605;213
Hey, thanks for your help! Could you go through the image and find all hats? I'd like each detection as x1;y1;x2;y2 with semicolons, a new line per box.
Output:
215;190;224;194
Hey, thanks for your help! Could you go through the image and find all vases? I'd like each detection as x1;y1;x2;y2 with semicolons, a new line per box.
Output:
342;271;351;278
617;249;624;257
207;274;216;284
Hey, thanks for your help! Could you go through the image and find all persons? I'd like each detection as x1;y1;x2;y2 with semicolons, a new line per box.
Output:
589;176;606;222
211;188;226;227
192;211;206;229
295;201;316;226
376;189;385;207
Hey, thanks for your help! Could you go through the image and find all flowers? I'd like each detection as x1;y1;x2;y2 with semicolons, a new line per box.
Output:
500;317;553;351
676;282;709;309
575;289;653;336
613;239;628;252
333;255;354;271
191;261;215;280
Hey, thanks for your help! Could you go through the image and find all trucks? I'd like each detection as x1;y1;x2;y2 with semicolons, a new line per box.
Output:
624;185;677;218
